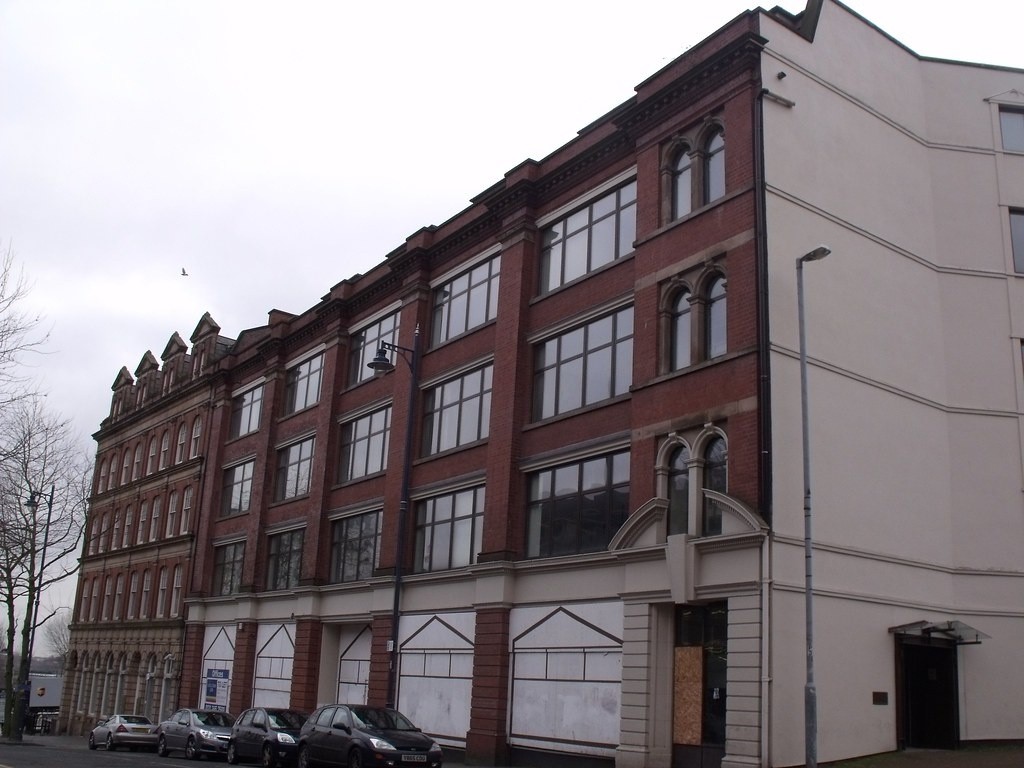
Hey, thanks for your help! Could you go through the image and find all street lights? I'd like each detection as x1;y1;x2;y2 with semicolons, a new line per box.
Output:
796;244;841;768
366;321;422;709
20;481;55;738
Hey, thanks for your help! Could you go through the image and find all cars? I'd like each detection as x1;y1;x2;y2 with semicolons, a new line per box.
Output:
295;702;444;768
227;707;312;768
87;714;159;753
157;707;238;761
